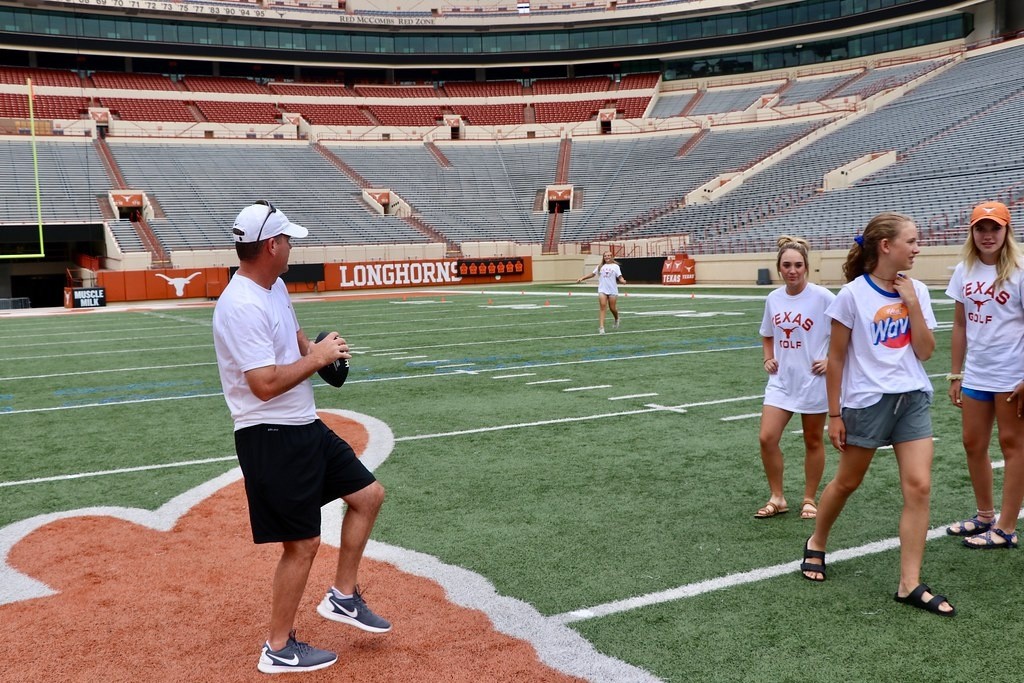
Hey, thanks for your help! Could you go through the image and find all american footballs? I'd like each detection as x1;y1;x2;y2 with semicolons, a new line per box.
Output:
315;331;349;389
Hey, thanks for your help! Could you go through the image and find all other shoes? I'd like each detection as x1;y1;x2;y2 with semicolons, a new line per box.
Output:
599;327;605;335
613;317;619;328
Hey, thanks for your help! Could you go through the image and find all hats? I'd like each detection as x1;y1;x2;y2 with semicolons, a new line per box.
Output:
232;203;309;243
970;200;1010;227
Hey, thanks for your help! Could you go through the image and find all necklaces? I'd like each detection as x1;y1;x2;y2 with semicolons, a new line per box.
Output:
870;272;894;281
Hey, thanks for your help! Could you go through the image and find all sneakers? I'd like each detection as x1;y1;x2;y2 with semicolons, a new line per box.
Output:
258;630;339;674
317;584;393;634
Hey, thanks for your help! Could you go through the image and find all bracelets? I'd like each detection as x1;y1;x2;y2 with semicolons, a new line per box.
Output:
764;359;771;364
829;414;840;418
947;373;964;380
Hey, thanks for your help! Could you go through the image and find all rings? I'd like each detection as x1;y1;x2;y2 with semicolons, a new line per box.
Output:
816;363;821;367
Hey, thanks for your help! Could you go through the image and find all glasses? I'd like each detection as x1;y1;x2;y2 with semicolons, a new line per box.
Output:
253;199;276;242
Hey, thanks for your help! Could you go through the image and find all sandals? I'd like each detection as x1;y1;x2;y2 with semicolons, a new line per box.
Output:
963;528;1018;549
946;515;997;536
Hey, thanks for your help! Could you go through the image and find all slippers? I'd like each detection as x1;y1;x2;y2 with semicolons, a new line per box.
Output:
800;501;817;519
753;502;789;517
894;583;955;617
801;536;827;582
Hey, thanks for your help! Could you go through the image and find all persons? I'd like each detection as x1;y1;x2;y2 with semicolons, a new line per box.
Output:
945;201;1024;548
575;251;626;335
213;200;393;673
801;212;955;617
754;235;836;519
